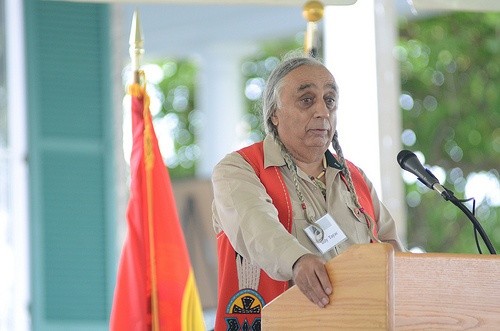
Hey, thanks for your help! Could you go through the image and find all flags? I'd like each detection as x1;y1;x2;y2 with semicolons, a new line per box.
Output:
109;75;208;331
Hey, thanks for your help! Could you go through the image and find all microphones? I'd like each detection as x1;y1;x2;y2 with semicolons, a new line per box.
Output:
396;149;451;202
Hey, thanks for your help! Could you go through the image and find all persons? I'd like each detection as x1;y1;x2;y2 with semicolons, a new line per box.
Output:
210;56;405;331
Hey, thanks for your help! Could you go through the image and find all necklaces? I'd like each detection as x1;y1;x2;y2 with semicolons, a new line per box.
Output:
307;153;330;199
